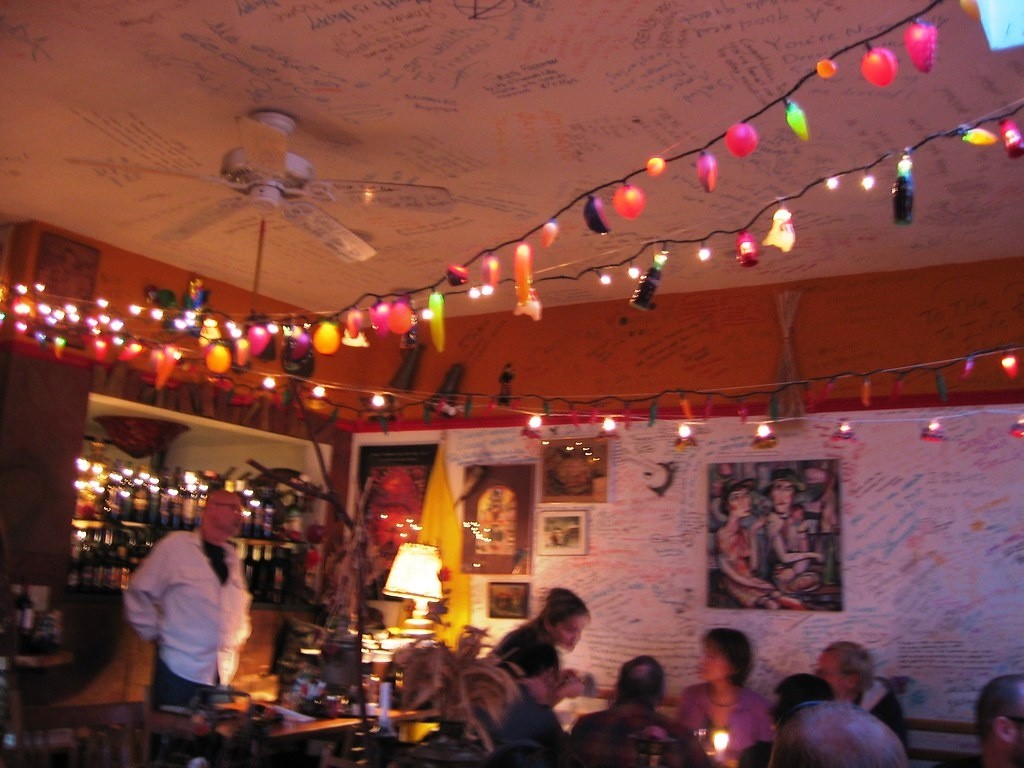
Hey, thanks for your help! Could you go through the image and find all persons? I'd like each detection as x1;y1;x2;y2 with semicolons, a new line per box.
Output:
736;642;1024;768
123;489;253;768
556;656;711;768
472;589;590;768
673;628;773;768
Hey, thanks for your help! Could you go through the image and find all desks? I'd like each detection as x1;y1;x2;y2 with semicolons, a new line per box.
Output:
253;706;442;768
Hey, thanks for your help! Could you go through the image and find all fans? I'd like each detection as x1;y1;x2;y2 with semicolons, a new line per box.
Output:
64;111;454;262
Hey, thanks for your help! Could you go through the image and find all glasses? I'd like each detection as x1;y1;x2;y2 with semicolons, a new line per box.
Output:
212;500;244;514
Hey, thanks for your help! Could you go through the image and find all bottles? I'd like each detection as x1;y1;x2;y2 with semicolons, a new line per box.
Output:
15;585;34;656
70;460;276;601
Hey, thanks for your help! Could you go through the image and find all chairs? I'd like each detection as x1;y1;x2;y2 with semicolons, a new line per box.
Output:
18;700;150;768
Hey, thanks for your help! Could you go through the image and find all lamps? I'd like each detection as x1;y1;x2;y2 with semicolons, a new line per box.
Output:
382;543;444;629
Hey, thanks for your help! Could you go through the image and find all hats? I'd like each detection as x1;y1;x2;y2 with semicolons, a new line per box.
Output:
777;673;833;703
